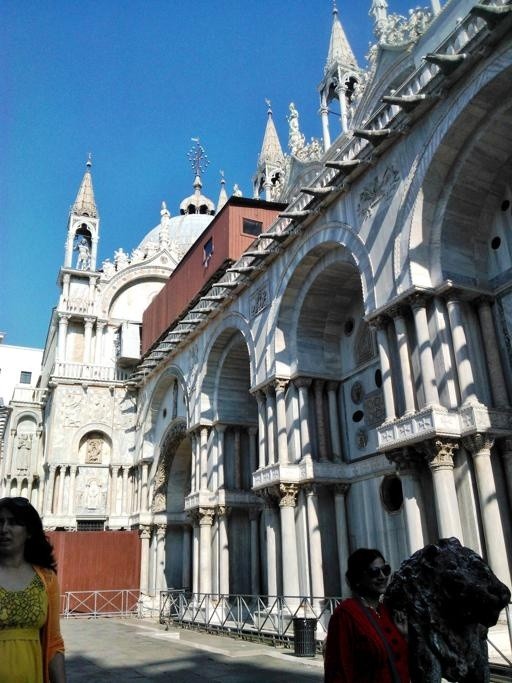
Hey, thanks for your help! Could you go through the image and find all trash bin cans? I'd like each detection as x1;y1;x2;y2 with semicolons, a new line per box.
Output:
292;617;317;657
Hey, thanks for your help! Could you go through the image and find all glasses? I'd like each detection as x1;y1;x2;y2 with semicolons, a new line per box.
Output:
367;565;391;578
0;497;29;507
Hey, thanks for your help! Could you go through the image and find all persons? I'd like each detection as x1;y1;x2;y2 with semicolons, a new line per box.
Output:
73;239;91;270
0;497;67;683
16;434;32;476
324;548;408;683
